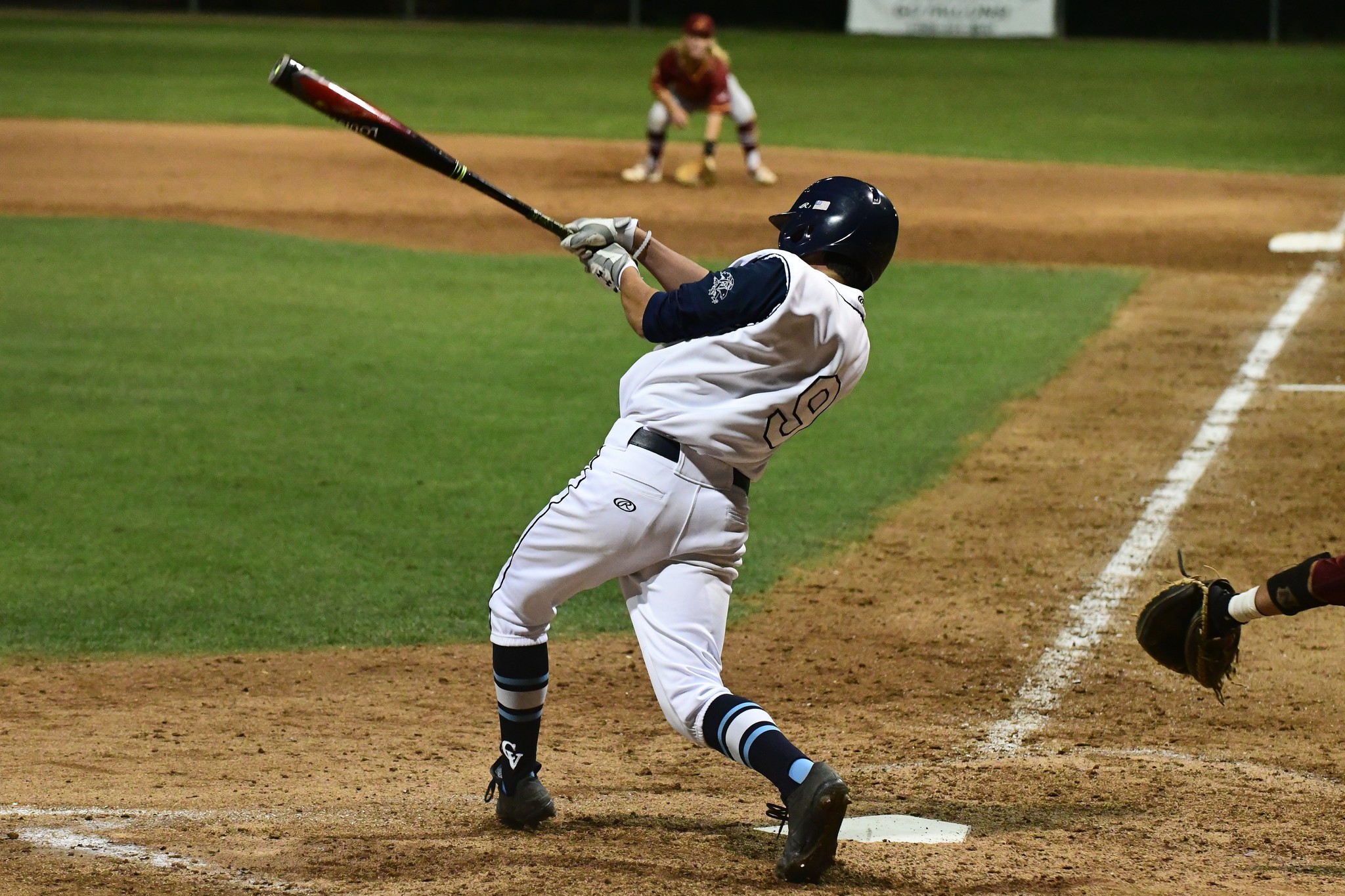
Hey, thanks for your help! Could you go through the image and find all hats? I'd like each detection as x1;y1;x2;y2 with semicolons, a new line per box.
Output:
685;15;714;39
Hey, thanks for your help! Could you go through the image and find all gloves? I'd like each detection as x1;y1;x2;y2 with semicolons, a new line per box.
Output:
560;215;639;255
567;239;639;292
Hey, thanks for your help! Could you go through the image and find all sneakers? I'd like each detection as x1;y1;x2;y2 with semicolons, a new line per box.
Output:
764;764;851;884
484;755;556;829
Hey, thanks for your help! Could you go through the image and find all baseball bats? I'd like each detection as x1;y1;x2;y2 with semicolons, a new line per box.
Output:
268;54;638;271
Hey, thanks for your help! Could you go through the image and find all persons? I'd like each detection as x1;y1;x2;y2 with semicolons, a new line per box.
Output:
621;15;777;185
480;176;897;878
1134;547;1345;706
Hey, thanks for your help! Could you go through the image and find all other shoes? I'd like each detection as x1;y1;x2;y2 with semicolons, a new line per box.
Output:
621;161;663;182
745;165;776;184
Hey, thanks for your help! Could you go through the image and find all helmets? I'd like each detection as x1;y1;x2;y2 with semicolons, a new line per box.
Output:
767;176;899;290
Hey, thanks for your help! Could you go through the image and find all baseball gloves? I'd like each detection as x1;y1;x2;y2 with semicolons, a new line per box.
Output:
1134;577;1242;690
673;150;721;191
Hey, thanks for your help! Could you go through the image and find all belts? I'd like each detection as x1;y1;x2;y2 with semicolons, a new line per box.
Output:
629;429;750;496
